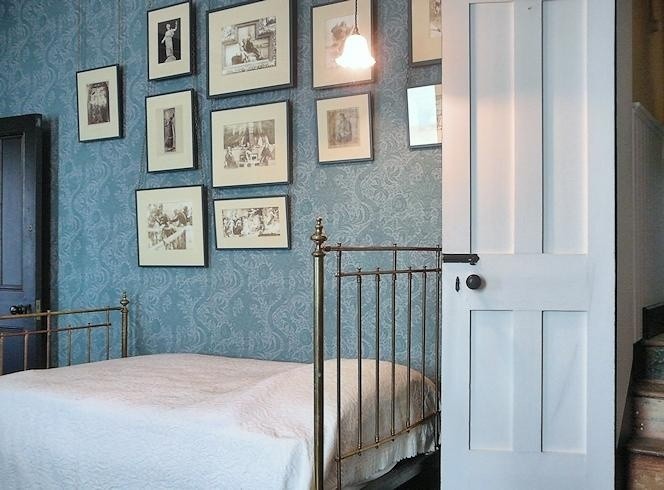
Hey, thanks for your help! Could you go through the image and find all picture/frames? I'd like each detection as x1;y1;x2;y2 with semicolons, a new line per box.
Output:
145;88;199;173
209;99;292;187
147;0;197;82
406;82;442;150
315;90;374;166
205;0;297;100
310;0;377;91
135;183;209;268
212;195;292;251
408;0;442;66
76;63;124;142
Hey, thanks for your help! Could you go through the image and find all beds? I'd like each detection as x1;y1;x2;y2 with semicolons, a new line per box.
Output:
0;217;442;490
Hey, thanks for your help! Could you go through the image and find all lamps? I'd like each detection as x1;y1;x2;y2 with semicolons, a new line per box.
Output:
335;0;377;73
